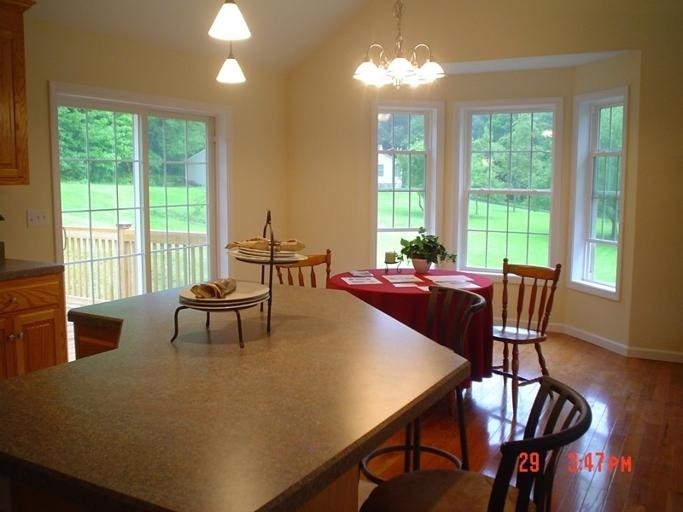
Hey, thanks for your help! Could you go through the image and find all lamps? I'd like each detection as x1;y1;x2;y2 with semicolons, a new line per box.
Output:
352;1;445;89
208;2;253;87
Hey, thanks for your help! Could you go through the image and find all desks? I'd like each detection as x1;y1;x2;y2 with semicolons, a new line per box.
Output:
327;268;493;416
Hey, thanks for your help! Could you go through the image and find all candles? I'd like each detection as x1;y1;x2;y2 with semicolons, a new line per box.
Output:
384;251;396;264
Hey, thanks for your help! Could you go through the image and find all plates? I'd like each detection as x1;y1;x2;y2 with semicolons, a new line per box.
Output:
177;281;270;313
225;247;309;265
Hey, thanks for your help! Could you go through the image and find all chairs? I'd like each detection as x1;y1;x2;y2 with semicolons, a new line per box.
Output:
274;248;331;291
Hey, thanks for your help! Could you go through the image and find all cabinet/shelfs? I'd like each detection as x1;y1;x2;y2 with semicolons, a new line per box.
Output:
0;7;31;187
0;273;66;381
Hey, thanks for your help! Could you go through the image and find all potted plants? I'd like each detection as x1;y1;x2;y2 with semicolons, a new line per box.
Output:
396;226;458;274
